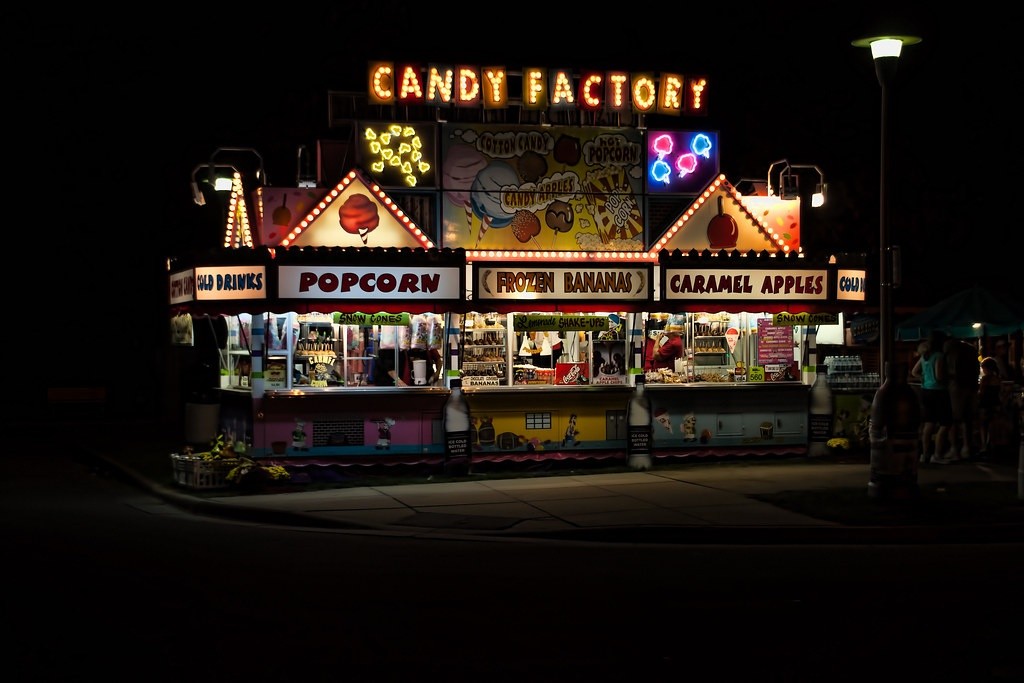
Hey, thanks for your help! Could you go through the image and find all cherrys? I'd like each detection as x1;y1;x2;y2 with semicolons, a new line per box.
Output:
272;193;292;225
707;195;738;248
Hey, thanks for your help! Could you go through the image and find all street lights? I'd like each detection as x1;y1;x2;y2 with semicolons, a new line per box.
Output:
851;32;922;386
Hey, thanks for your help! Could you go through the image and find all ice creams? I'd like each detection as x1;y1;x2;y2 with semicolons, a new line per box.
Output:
725;328;739;354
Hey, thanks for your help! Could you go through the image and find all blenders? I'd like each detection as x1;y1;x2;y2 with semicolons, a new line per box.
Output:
410;359;427;385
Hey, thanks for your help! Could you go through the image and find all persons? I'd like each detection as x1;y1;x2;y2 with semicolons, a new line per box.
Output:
373;323;1002;465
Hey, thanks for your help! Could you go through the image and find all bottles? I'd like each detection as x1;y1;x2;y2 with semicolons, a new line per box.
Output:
442;378;472;477
867;360;920;505
830;355;864;374
808;365;832;459
829;371;882;390
183;362;221;453
626;374;656;472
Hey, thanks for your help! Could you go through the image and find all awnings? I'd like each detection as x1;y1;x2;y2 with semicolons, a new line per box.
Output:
267;243;467;316
472;260;655;312
655;247;830;314
166;243;272;318
829;260;867;309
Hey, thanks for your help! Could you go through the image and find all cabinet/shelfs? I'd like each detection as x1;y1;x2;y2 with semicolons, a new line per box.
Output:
589;317;628;385
228;314;292;390
459;313;507;381
692;313;730;382
293;316;343;387
